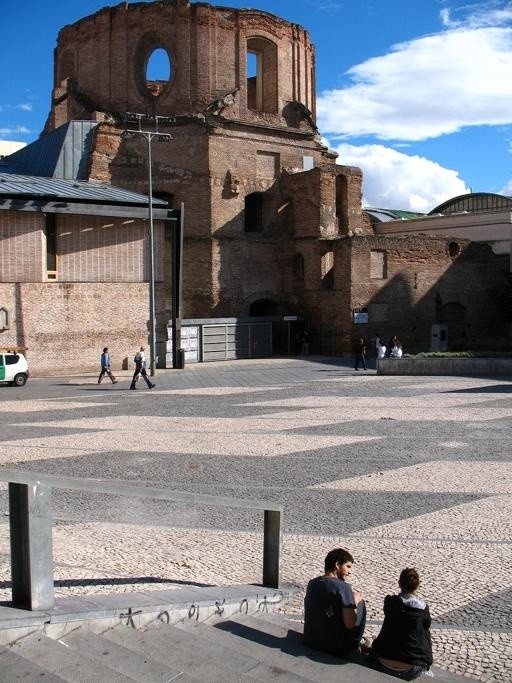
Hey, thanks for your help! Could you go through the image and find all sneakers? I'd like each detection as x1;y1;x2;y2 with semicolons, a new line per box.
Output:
149;383;156;389
113;381;118;384
129;388;137;390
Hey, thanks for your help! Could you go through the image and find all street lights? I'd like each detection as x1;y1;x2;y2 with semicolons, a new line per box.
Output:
120;112;177;376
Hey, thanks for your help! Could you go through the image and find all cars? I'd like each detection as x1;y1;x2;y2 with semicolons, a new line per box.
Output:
0;352;31;387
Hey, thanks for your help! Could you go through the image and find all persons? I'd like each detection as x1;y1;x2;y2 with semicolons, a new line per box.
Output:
130;345;156;389
98;347;119;384
304;549;435;681
293;329;310;357
354;336;403;370
